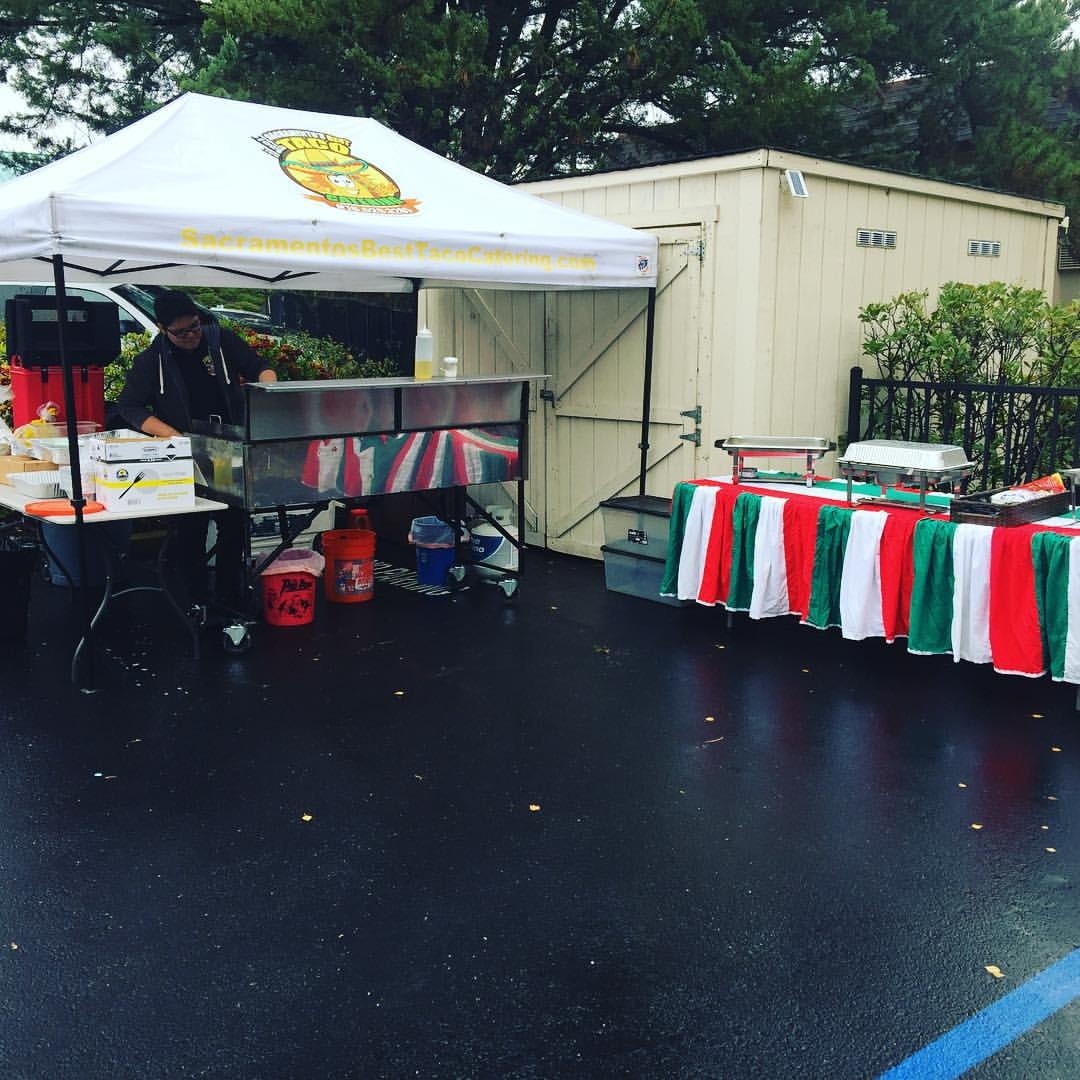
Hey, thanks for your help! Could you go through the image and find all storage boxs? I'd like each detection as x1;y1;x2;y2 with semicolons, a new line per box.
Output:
600;543;695;607
949;484;1071;529
11;358;107;435
600;494;673;558
0;455;58;485
89;436;195;513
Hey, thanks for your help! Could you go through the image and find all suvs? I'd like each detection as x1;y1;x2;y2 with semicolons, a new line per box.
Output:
0;282;269;343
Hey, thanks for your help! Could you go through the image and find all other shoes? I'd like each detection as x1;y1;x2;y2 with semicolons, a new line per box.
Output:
222;615;252;656
185;598;208;625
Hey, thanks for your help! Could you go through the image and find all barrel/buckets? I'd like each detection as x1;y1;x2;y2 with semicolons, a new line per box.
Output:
408;515;456;586
320;528;376;603
256;547;325;626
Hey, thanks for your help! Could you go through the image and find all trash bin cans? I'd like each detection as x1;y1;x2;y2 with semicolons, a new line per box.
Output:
412;516;464;586
259;547;318;627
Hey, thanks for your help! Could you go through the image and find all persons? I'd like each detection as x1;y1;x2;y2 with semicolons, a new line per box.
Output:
118;291;276;622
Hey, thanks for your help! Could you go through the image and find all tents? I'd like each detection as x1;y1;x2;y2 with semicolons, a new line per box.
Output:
0;91;658;692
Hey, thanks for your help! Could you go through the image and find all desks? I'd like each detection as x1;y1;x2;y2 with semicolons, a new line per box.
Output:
660;470;1080;683
0;485;229;695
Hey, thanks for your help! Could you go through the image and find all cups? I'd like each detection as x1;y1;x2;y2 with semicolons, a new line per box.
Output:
443;357;458;377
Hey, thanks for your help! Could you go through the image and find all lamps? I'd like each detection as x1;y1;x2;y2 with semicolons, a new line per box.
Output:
1059;215;1069;228
780;168;808;197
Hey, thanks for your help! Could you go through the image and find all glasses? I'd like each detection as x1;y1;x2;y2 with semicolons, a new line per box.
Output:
165;321;201;338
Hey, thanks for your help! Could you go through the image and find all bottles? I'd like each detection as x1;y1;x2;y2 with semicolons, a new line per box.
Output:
415;323;432;379
